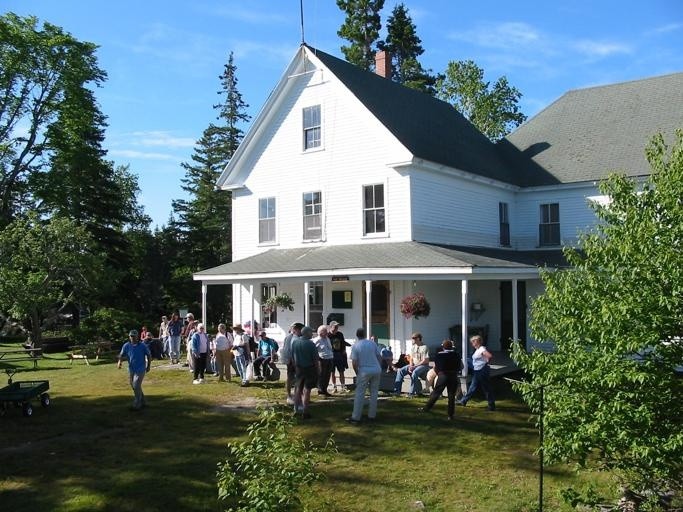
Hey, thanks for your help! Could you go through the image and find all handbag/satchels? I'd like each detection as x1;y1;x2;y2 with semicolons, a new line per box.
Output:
395;353;409;368
268;362;280;380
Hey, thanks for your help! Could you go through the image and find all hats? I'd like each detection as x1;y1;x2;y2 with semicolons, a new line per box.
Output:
229;323;246;332
329;320;340;327
128;329;139;337
183;312;195;319
146;332;152;335
300;326;316;335
440;341;452;349
411;332;422;338
258;331;266;336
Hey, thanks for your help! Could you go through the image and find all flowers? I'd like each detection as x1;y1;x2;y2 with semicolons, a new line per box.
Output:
399;289;431;320
265;292;295;312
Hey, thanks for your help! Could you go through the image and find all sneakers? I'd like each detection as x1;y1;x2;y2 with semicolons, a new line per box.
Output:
392;386;431;397
182;361;193;373
192;377;208;384
128;399;145;409
317;389;335;399
285;396;296;405
213;372;232;383
291;411;313;420
455;401;466;406
332;385;351;394
343;416;376;425
240;378;250;387
417;405;428;414
254;375;268;382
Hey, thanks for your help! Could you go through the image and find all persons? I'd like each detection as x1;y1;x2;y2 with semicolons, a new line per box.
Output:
388;330;429;398
116;329;151;412
141;331;154;348
455;335;496;412
138;325;154;341
421;339;462;400
416;340;463;421
157;312;398;427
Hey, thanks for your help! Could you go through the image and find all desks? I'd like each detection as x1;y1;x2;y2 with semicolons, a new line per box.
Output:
0;348;42;368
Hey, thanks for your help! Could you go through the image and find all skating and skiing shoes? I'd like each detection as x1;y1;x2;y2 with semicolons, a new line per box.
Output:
171;359;181;365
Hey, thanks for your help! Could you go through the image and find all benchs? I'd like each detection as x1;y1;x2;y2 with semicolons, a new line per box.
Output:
0;357;41;371
65;340;122;366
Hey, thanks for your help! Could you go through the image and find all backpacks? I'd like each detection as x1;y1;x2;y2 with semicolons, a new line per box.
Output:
268;337;279;353
240;333;258;353
